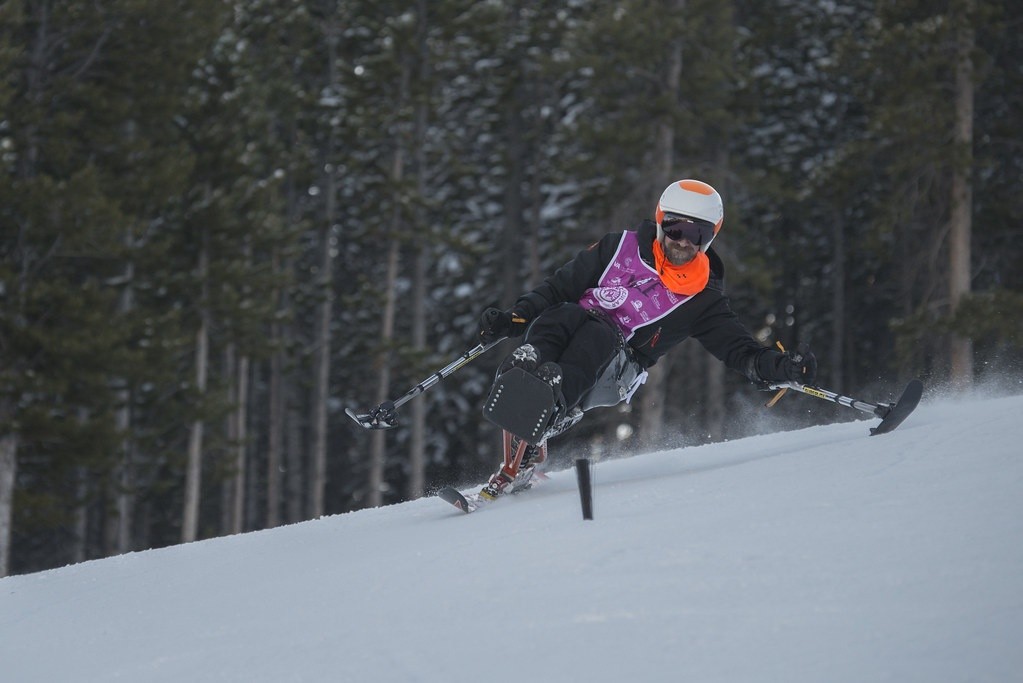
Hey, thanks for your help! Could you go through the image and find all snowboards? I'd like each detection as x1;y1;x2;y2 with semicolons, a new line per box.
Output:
436;481;534;514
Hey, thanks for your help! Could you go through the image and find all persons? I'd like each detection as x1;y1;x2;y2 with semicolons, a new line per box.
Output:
478;179;818;430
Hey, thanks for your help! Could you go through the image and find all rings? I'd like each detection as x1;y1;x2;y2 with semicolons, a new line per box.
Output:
481;331;484;335
803;366;806;374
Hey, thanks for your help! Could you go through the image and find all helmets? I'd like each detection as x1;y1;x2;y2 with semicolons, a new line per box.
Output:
655;179;723;252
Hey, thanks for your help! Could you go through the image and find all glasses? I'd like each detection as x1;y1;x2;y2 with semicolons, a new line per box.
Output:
662;213;715;245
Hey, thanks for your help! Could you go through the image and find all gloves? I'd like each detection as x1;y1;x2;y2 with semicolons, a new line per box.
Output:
783;348;817;387
477;307;511;346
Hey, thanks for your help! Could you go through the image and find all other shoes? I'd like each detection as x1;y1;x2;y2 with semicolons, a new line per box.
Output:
532;361;564;407
495;343;541;374
